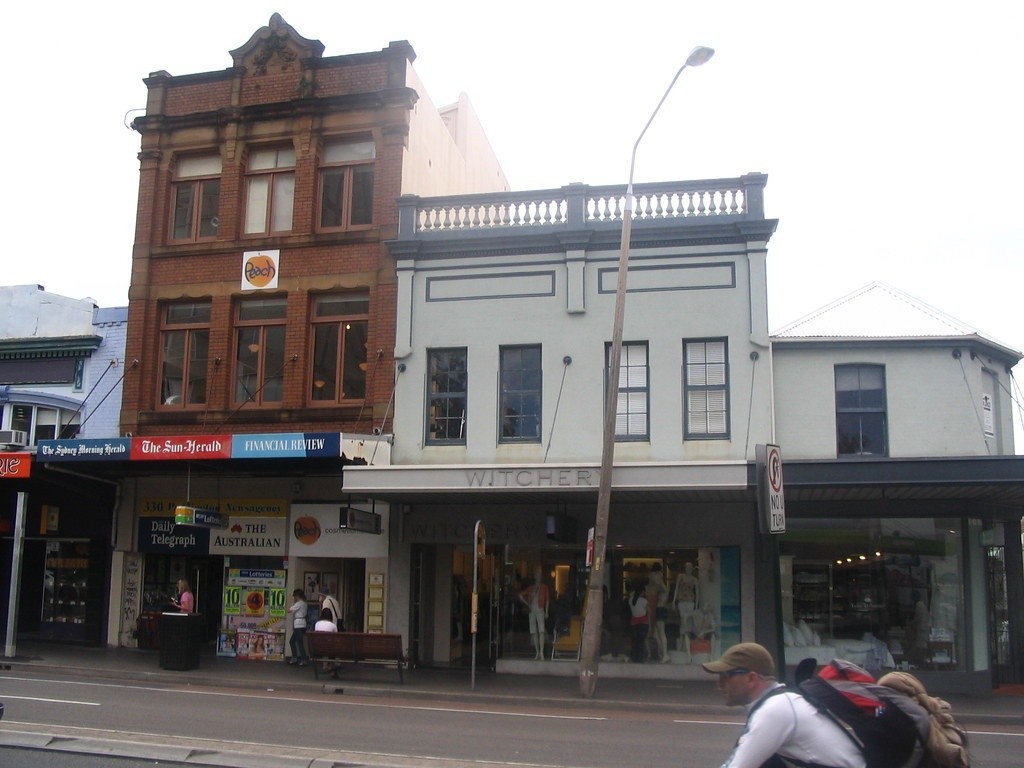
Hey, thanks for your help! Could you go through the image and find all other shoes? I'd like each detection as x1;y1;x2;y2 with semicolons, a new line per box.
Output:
299;661;307;666
289;658;297;664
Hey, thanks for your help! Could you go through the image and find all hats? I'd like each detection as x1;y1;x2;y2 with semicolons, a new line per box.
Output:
293;589;304;596
318;587;329;596
702;642;774;675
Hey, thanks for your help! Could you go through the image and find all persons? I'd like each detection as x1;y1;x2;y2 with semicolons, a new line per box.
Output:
653;569;670;664
171;579;194;613
906;590;929;658
629;584;652;663
682;599;717;664
518;573;550;660
700;642;872;768
320;587;343;632
249;636;266;659
287;589;308;665
315;608;342;671
671;563;699;665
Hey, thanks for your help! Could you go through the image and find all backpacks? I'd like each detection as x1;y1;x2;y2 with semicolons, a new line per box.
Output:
745;658;930;768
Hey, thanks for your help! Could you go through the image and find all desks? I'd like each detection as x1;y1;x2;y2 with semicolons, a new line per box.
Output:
928;641;954;668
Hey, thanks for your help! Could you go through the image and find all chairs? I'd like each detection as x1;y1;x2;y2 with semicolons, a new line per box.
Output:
551;615;583;661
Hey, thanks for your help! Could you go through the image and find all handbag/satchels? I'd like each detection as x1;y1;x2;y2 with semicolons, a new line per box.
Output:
337;619;346;632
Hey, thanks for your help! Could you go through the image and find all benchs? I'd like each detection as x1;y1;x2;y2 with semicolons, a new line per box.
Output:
307;631;407;684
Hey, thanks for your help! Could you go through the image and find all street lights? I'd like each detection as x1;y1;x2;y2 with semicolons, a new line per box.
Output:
572;45;717;705
